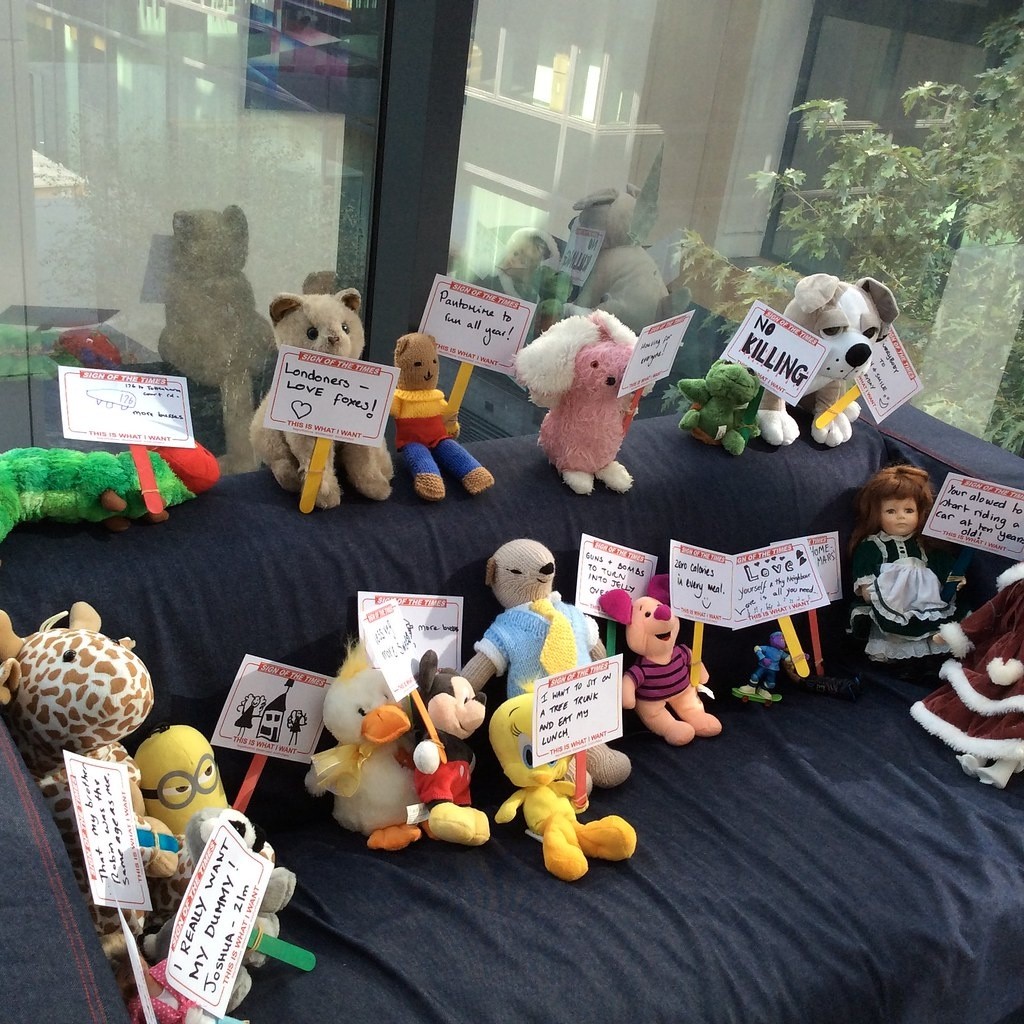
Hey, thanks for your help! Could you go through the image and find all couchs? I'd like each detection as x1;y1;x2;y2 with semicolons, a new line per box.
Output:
0;394;1024;1023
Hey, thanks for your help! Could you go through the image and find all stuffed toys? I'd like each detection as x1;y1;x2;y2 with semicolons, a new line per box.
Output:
492;187;693;347
1;328;126;389
678;359;759;456
756;275;899;447
144;811;295;1011
462;539;631;794
134;723;234;844
2;598;276;953
510;308;656;494
306;637;435;853
489;692;636;880
599;574;721;745
159;202;279;475
2;434;220;539
249;287;395;508
410;650;491;845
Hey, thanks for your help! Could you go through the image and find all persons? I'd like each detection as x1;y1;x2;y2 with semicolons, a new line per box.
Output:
847;466;968;660
740;631;810;699
921;557;1024;786
383;332;495;497
98;932;250;1024
